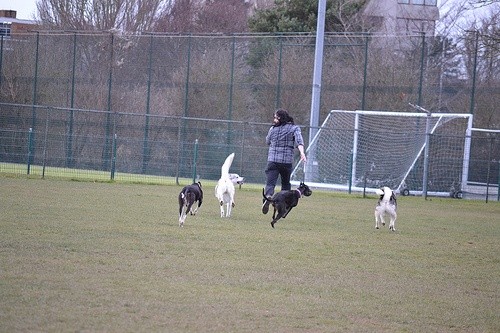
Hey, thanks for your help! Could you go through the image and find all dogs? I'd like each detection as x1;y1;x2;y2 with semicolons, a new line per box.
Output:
263;181;312;228
214;153;245;218
375;186;398;232
178;182;203;227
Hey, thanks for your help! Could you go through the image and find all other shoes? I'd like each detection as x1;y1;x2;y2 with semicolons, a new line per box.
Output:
262;196;272;214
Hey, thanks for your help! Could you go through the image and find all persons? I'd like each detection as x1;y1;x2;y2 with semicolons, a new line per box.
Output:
262;110;307;214
